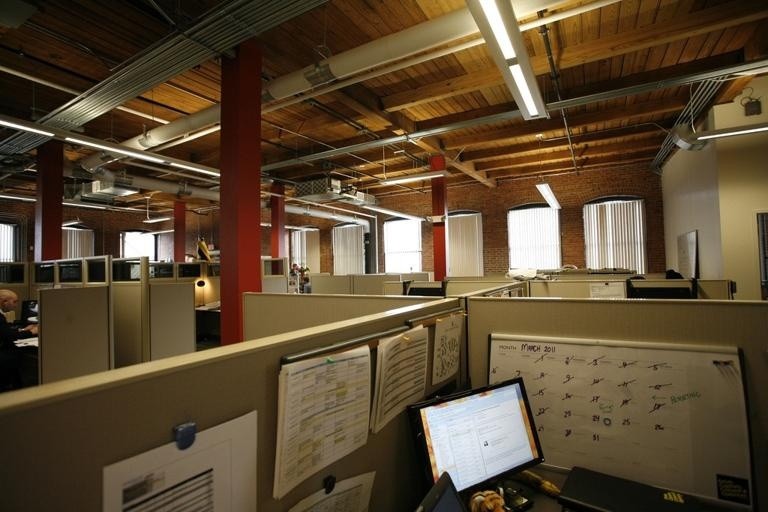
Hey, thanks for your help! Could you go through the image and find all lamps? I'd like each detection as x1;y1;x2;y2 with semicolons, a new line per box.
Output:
197;281;206;307
377;143;448;186
534;134;563;210
0;114;220;178
140;199;174;236
689;81;768;142
62;214;83;227
466;0;551;124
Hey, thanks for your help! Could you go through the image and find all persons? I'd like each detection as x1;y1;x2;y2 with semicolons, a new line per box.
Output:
299;276;311;293
290;263;300;276
0;288;40;392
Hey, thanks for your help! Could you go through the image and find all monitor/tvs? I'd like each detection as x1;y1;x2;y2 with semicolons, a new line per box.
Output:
128;263;156;280
406;376;545;512
413;472;471;512
20;300;39;329
